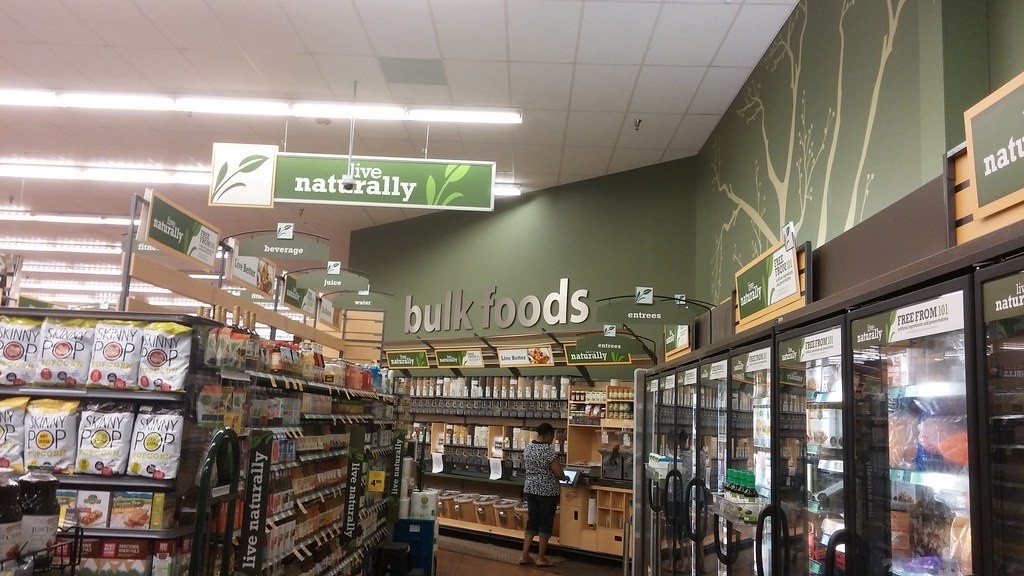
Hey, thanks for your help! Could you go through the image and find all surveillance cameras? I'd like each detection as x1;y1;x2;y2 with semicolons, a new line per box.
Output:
342;174;354;190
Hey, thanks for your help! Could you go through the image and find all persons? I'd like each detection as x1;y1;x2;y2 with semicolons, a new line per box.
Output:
664;429;707;574
519;423;570;566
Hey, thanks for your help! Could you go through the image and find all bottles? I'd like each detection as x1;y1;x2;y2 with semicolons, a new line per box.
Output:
571;391;576;400
0;467;23;568
576;391;580;401
18;465;60;569
724;468;759;525
581;391;585;401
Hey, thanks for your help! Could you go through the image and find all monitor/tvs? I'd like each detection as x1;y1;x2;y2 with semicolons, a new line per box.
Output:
558;469;581;486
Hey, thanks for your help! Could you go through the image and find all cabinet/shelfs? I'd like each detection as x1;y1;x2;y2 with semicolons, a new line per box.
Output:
0;307;658;576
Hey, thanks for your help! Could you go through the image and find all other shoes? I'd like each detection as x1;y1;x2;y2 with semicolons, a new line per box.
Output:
519;557;535;564
536;559;555;567
